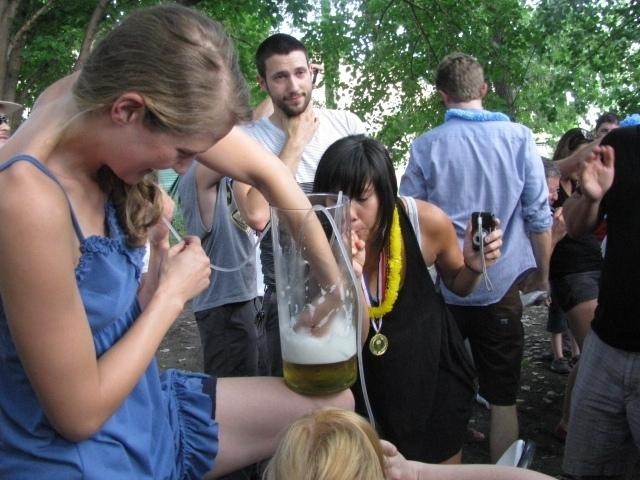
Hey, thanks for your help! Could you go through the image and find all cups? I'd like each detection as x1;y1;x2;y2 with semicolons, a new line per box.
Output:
269;191;361;399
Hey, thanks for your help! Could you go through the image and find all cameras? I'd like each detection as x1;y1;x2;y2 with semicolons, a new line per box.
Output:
472;211;494;251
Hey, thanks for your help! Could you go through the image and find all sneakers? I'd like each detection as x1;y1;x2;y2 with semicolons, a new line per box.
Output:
551;358;569;374
